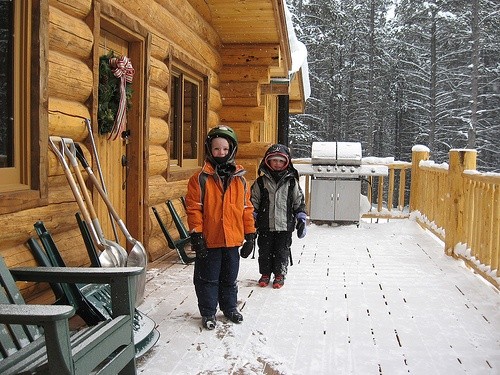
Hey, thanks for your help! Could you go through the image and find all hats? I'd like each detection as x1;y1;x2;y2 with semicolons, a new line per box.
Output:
267;154;287;163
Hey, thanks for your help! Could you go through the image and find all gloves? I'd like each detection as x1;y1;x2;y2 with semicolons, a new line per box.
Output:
189;231;207;259
240;233;257;258
296;211;307;239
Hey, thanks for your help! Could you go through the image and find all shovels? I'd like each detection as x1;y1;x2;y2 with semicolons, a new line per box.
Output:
49;136;148;308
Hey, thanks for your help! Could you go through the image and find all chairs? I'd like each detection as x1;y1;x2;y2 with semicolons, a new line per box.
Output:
0;196;196;375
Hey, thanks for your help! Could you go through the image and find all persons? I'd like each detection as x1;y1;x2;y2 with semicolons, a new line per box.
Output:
248;144;307;289
185;125;257;331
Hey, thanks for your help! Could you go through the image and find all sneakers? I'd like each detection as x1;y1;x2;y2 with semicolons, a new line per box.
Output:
202;314;217;329
224;308;243;323
259;272;271;287
272;274;285;289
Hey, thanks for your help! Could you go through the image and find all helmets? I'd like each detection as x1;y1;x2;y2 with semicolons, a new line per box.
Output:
205;124;238;146
